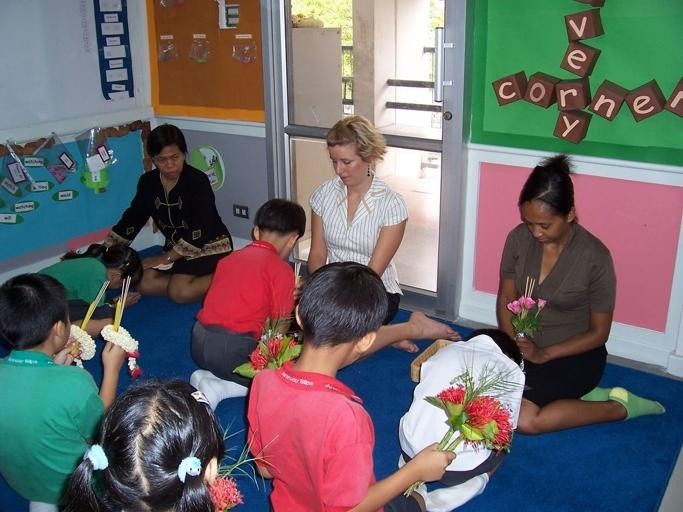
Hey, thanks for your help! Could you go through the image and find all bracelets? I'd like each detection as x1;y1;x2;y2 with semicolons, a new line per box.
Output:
164;252;174;264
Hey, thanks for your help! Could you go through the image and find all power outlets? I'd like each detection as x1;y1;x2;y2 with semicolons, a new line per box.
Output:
232;203;248;219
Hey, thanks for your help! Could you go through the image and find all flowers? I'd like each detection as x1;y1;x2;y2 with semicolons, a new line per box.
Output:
504;294;548;338
201;411;284;512
229;304;303;385
403;344;526;500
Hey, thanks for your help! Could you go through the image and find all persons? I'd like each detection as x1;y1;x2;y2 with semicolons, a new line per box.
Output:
55;374;225;512
245;261;457;512
189;199;307;411
396;327;527;512
102;124;234;305
38;243;144;322
0;272;126;505
306;115;409;327
495;155;666;436
